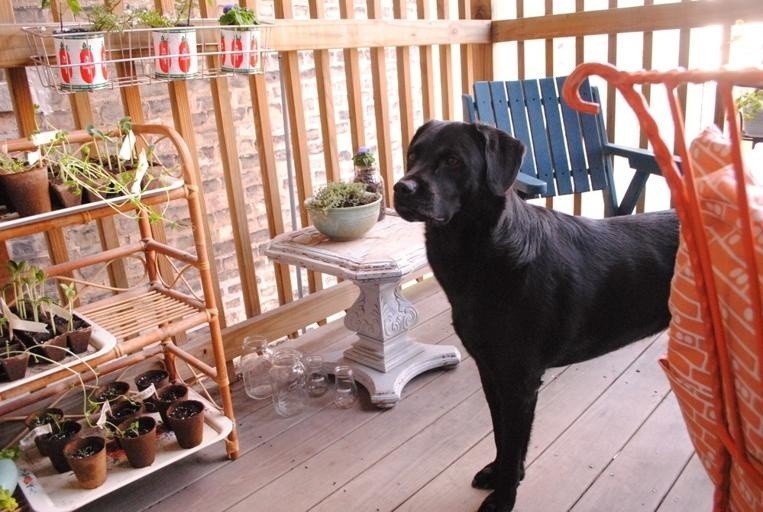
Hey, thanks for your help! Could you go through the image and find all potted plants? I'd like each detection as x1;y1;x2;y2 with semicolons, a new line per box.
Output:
736;87;763;140
303;184;380;239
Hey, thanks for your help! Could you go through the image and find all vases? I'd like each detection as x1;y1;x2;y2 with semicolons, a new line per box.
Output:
221;26;261;72
355;167;387;220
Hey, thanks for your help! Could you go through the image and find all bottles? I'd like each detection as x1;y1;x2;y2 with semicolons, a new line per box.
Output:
240;336;274;400
333;366;359;410
303;355;329;397
268;348;308;419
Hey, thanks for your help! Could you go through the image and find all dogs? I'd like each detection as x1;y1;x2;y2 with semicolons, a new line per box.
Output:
392;118;681;512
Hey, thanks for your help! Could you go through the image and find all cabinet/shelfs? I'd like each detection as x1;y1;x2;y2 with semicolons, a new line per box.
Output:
1;122;239;512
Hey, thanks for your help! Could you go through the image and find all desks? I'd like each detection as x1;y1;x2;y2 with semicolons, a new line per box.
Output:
263;207;462;411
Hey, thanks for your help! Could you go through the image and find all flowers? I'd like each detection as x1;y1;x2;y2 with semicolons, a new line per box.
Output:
353;146;375;168
220;4;255;26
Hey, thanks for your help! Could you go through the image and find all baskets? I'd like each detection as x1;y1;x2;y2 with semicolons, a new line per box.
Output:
20;20;277;95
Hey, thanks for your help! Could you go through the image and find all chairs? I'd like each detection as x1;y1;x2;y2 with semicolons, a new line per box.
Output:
461;75;683;218
